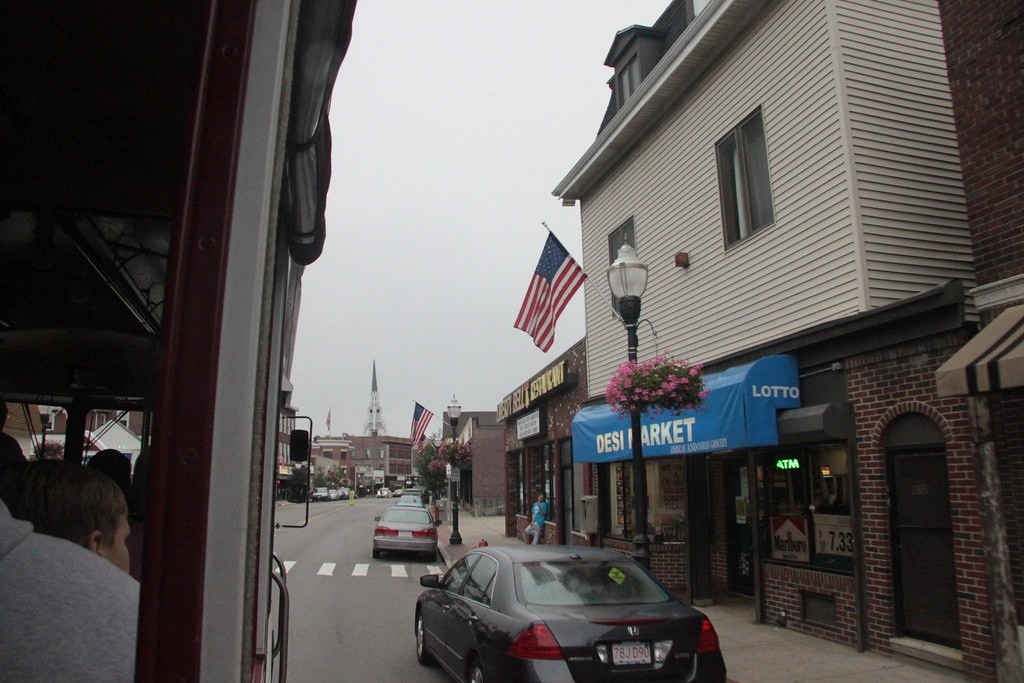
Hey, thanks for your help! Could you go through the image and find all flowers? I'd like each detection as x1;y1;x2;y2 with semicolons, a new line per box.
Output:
605;347;710;421
438;440;478;468
427;460;446;476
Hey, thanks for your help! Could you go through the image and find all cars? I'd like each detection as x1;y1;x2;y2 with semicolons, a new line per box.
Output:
313;487;350;502
370;485;442;563
413;543;726;683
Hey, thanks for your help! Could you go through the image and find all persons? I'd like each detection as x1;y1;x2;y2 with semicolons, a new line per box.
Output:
0;401;146;582
525;492;547;545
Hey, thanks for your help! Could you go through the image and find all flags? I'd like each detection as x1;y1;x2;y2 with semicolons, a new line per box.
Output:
326;412;330;431
410;402;433;445
417;434;430;453
514;231;588;353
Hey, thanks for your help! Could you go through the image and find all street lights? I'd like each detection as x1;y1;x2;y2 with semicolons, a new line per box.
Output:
607;229;647;577
446;394;462;544
343;468;347;487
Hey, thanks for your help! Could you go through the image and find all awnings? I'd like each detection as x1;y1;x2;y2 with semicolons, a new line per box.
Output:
934;304;1024;398
572;355;802;464
